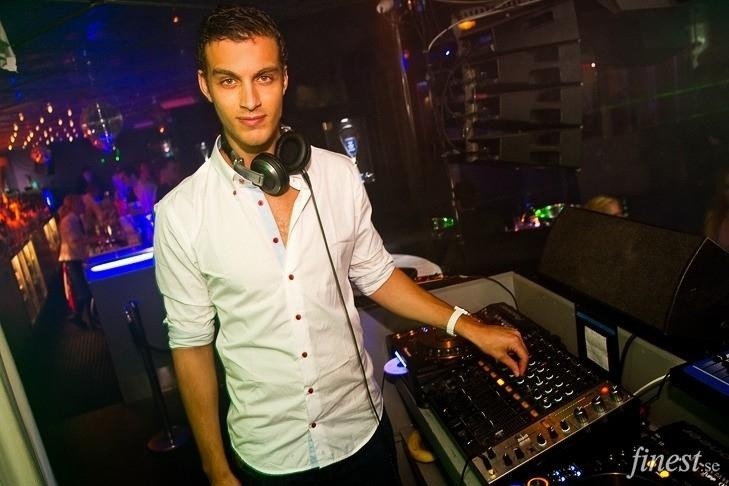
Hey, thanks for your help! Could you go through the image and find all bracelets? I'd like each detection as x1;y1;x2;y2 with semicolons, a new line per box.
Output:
446;305;471;338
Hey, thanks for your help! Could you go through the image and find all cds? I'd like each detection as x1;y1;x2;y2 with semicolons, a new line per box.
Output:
383;355;409;375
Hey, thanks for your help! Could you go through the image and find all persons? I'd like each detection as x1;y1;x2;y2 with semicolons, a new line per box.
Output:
152;3;529;486
57;161;182;328
585;194;630;218
703;165;729;249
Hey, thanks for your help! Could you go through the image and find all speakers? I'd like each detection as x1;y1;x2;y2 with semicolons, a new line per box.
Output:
538;206;729;340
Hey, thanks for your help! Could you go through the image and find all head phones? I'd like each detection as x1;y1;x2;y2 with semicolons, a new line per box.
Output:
220;118;310;196
